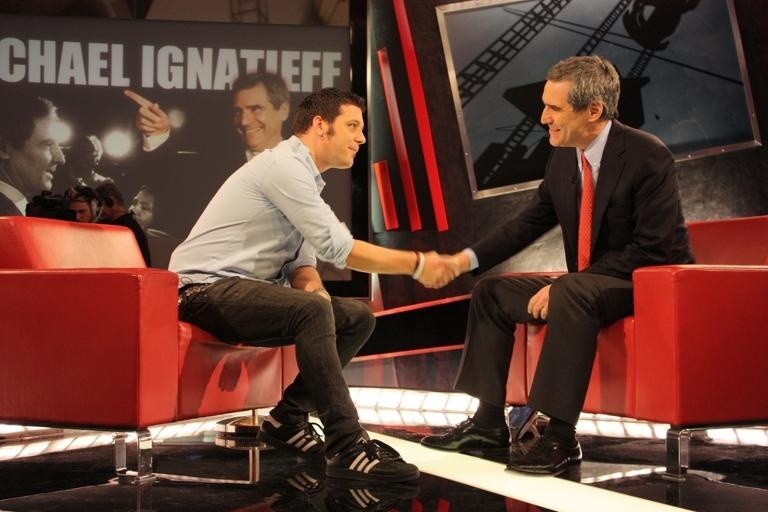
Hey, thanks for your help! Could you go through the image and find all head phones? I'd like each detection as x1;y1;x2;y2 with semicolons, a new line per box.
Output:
75;186;101;217
96;188;114;207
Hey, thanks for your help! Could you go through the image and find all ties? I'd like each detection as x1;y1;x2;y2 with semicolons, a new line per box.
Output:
575;149;598;276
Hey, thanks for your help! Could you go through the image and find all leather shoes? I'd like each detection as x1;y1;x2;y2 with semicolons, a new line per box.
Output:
418;420;511;453
502;423;587;475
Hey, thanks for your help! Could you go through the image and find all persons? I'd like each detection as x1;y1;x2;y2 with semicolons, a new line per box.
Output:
121;72;293;248
57;132;114;192
0;93;65;217
62;185;96;223
126;185;172;243
167;87;456;488
94;183;153;269
424;52;697;476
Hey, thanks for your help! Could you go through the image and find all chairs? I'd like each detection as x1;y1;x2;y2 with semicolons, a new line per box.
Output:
497;215;768;483
0;214;303;491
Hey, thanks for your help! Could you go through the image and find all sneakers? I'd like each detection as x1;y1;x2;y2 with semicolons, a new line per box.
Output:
255;403;328;464
324;442;424;486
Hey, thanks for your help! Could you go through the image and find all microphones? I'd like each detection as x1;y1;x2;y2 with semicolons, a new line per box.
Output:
92;206;104;223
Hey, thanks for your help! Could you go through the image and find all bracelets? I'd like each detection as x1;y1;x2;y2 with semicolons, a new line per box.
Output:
411;250;424;280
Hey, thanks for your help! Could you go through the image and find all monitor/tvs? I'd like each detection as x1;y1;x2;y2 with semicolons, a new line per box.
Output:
0;0;372;302
